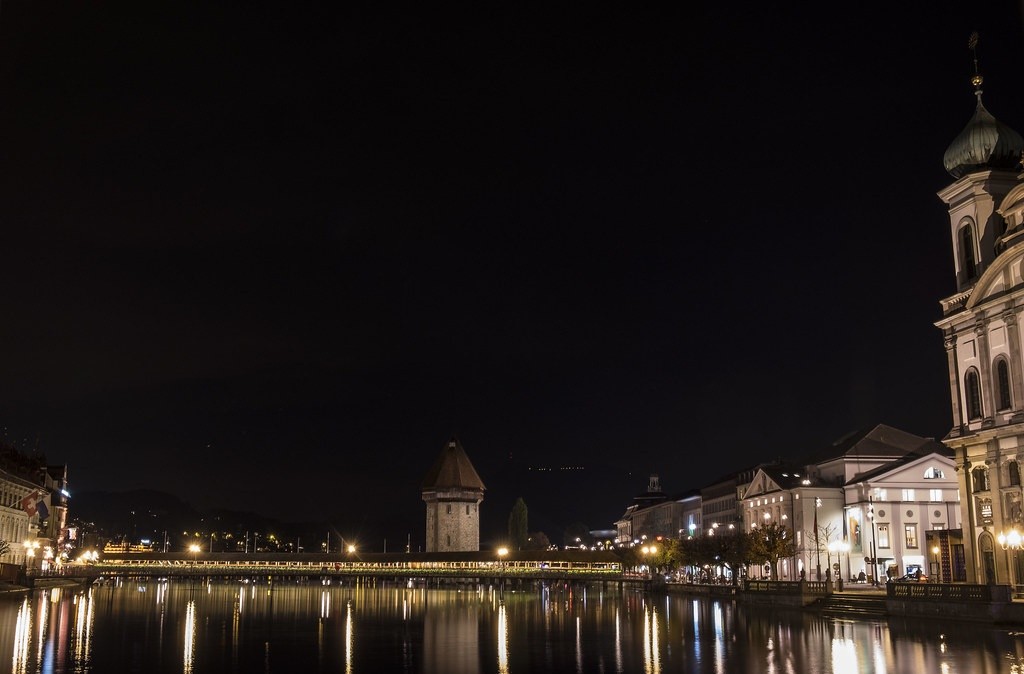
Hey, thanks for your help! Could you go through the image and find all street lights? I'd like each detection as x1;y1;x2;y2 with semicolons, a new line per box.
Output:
867;496;879;587
932;546;941;584
999;529;1024;599
641;545;656;579
830;541;846;592
814;497;822;581
497;546;507;568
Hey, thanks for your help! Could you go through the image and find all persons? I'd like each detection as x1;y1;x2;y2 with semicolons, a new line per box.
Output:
858;570;866;583
916;567;922;578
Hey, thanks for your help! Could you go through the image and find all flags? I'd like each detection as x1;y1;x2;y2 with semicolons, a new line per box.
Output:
20;492;39;519
36;494;51;520
